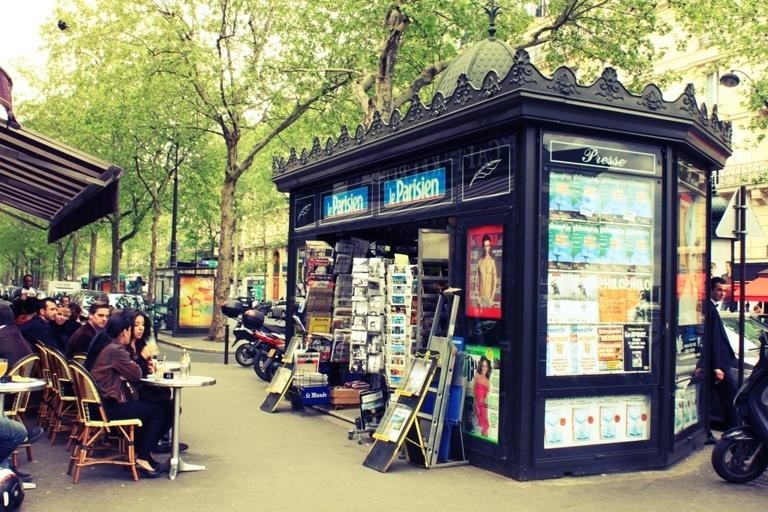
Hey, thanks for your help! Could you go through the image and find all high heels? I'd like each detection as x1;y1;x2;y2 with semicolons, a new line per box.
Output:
134;462;162;480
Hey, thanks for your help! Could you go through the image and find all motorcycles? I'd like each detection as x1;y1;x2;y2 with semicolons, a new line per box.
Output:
241;309;286;383
220;299;285;367
711;330;768;484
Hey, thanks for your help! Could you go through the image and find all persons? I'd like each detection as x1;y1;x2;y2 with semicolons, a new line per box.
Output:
706;277;741;444
474;357;492;437
474;234;497;307
749;305;763;315
125;308;158;378
0;304;31;373
711;262;717;280
67;303;82;340
60;296;70;307
55;304;68;326
21;297;65;356
12;274;37;302
85;312;163;477
65;302;107;355
721;261;732;312
15;297;39;324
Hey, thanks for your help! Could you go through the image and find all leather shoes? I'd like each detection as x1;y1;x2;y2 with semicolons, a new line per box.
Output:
703;437;720;443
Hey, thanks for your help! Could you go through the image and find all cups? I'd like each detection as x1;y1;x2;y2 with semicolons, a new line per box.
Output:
154;361;165;380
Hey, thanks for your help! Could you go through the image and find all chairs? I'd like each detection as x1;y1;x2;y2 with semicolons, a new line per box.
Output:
35;338;75;430
4;351;41;469
65;355;126;453
44;346;104;445
66;360;142;484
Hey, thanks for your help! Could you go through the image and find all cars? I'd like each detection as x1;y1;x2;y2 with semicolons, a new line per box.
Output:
267;300;287;320
708;309;768;424
71;289;159;360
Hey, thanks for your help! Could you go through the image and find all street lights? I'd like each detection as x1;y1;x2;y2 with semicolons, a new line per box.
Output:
720;69;768;115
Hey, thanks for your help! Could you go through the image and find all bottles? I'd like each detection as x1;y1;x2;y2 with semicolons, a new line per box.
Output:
187;352;191;376
181;349;189;380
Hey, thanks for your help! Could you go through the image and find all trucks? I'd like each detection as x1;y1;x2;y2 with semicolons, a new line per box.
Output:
46;280;82;300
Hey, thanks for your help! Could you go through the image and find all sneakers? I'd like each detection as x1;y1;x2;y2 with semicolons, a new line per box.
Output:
9;466;33;483
27;426;44;444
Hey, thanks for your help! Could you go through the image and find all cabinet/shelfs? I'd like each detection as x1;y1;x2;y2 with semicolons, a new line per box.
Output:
382;263;459;352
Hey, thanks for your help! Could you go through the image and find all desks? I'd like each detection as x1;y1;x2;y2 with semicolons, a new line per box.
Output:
142;373;216;481
155;358;192;453
0;377;47;416
